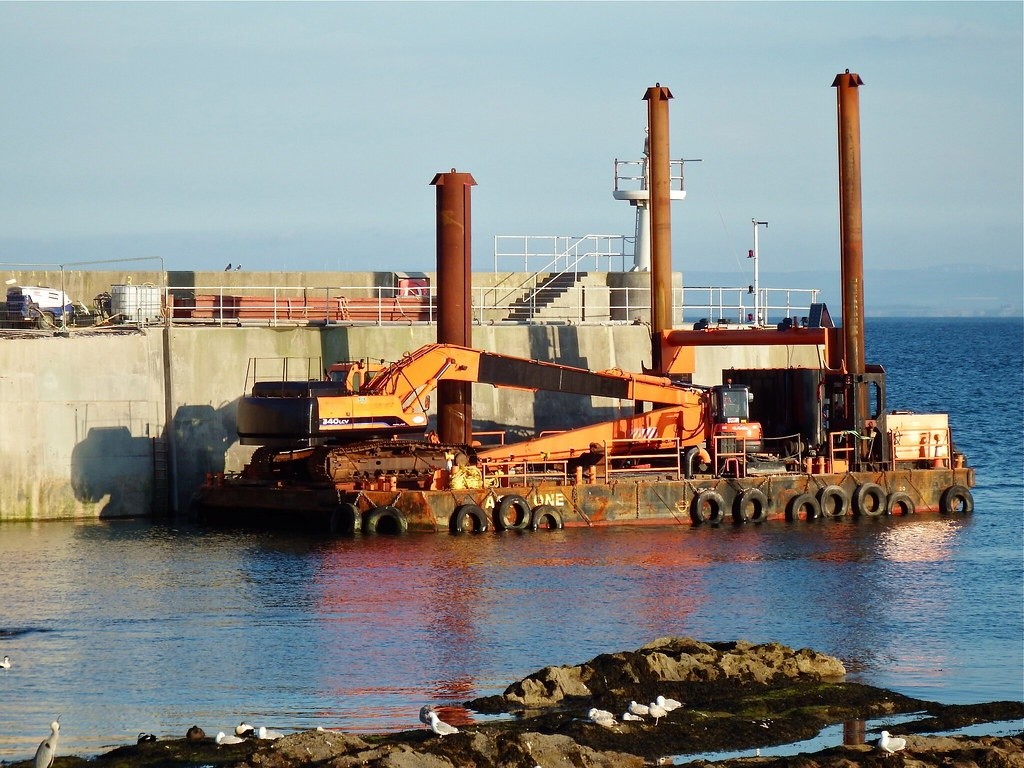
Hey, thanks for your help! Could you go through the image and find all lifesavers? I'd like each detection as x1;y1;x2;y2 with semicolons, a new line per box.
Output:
789;485;849;519
450;504;488;533
494;495;563;530
692;490;726;524
852;482;915;516
329;502;408;534
940;485;974;512
735;488;768;522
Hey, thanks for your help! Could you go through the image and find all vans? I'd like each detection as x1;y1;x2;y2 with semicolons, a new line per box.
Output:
7;285;75;329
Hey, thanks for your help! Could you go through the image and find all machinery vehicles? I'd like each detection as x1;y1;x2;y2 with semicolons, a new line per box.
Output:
201;343;763;494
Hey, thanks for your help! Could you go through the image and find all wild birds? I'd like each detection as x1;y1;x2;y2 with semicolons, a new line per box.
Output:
0;655;12;670
214;720;284;745
418;704;460;739
877;730;908;758
623;695;683;727
225;263;242;271
587;707;619;729
185;725;206;739
34;715;61;768
136;731;159;745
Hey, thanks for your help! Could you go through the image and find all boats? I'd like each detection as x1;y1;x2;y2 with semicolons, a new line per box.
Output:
191;70;979;535
